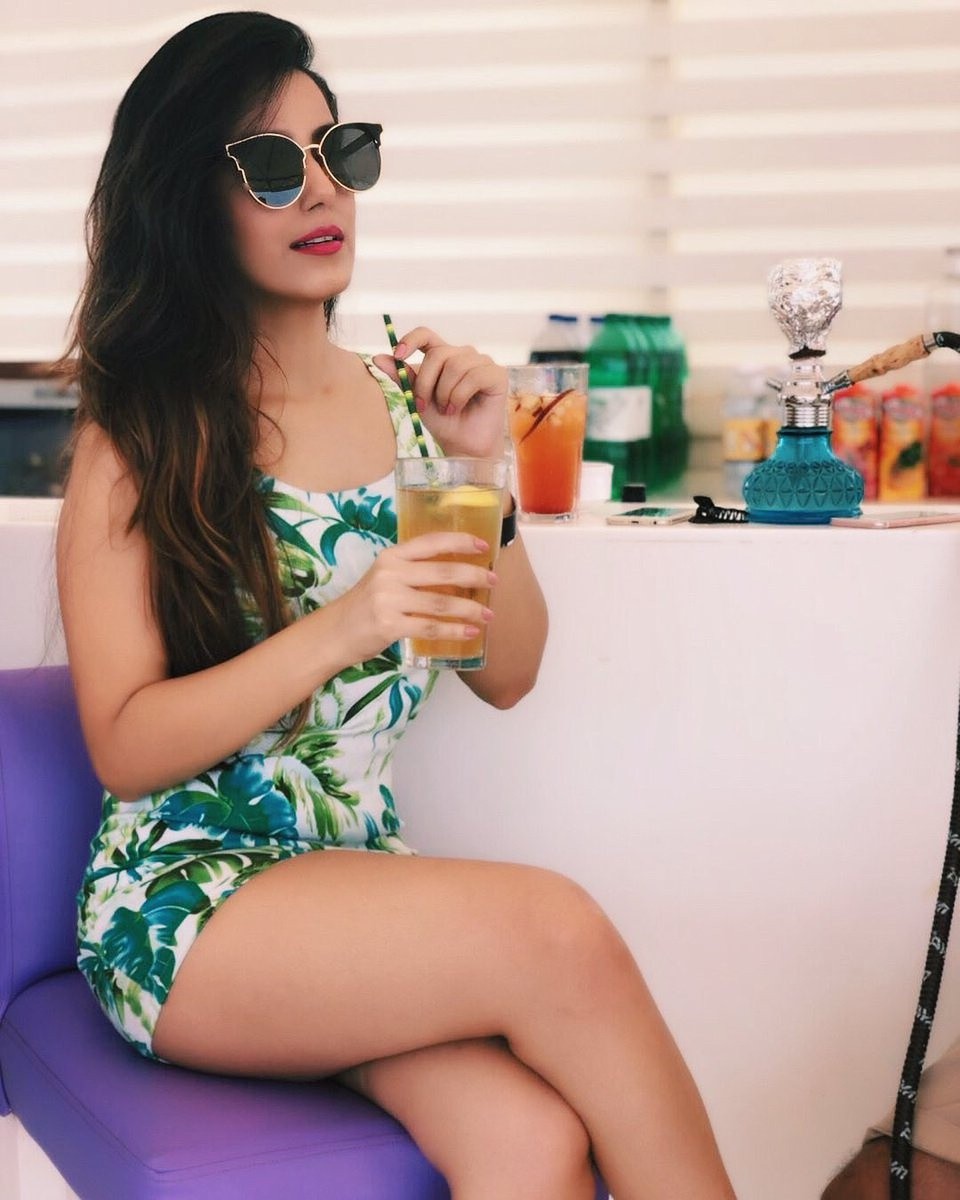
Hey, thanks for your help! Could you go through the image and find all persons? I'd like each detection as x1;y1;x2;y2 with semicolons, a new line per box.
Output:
57;10;735;1200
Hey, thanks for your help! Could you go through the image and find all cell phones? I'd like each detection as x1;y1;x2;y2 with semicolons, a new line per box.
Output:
606;508;697;526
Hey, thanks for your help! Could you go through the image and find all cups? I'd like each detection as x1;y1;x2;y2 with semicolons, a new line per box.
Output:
505;365;588;519
393;454;506;667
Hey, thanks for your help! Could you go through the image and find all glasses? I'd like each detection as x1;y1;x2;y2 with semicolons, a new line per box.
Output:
225;122;383;209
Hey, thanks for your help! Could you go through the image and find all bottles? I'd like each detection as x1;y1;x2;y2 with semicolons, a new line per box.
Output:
724;381;960;505
743;362;864;524
529;313;690;498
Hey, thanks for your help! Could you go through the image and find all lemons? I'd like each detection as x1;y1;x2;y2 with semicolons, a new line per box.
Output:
434;484;500;506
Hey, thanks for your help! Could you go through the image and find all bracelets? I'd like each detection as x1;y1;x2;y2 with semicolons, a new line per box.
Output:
501;495;517;548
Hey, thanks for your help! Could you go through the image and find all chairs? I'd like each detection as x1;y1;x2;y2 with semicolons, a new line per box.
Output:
0;665;455;1200
